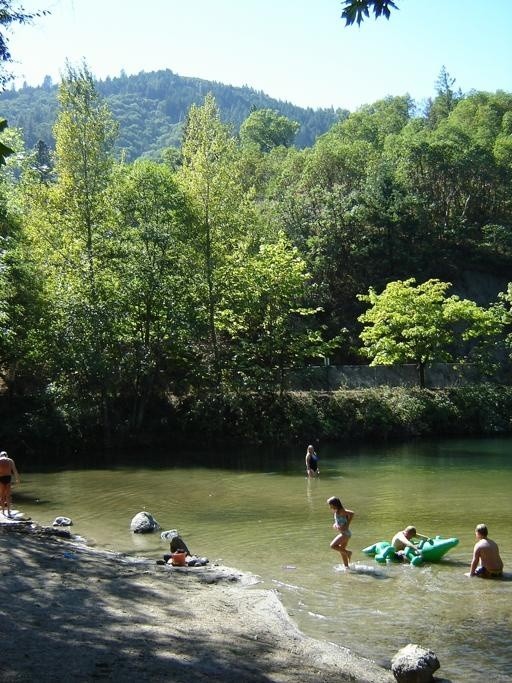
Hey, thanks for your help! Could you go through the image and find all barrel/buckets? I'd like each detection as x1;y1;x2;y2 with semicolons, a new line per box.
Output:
170;551;187;566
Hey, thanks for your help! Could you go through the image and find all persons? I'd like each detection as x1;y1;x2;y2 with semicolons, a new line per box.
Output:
465;523;503;578
391;525;429;564
305;444;320;478
0;450;20;517
326;496;355;567
